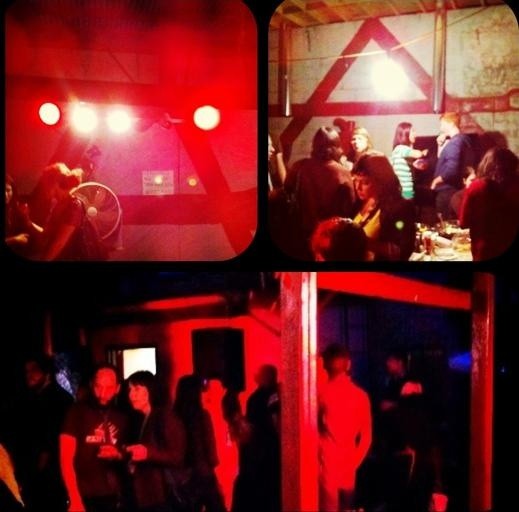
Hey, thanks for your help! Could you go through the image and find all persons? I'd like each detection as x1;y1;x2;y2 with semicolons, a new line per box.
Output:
5;172;35;260
267;107;519;261
17;160;111;261
1;344;471;511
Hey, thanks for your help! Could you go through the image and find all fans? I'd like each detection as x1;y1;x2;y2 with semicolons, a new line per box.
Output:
69;181;121;240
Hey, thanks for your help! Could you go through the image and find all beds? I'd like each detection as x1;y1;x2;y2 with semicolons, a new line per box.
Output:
409;246;473;262
